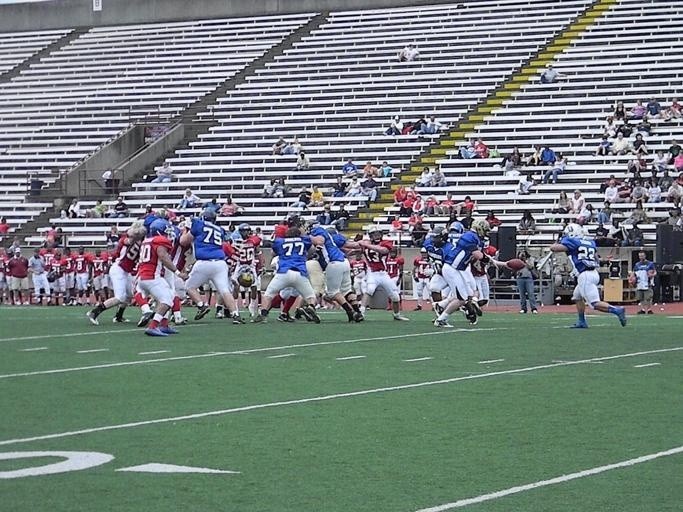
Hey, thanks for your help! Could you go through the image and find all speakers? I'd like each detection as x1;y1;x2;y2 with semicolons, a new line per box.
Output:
631;250;653;272
671;231;683;264
656;224;673;264
497;226;516;279
486;231;497;278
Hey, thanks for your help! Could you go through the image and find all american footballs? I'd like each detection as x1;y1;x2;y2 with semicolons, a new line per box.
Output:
647;270;655;276
507;259;525;269
630;276;636;284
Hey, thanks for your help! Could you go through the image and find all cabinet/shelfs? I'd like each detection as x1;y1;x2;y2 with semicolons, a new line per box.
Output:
603;276;645;304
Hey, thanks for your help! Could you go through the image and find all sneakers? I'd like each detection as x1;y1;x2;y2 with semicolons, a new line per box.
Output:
195;302;210;320
277;304;321;323
137;309;188;336
393;313;410;321
112;316;130;323
570;321;587;328
432;297;482;327
86;309;100;325
215;304;268;324
345;304;366;323
617;306;626;326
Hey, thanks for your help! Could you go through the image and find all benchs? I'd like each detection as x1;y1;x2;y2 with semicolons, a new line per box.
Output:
485;275;555;306
0;2;683;254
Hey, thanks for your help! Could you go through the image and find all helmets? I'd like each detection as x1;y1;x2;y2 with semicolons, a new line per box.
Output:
564;223;584;238
430;227;448;247
199;208;216;224
449;222;464;234
471;219;490;240
275;215;319;237
368;226;383;243
150;219;171;238
239;223;249;231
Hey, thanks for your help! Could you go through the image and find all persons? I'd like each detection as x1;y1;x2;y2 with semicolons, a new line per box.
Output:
151;162;173;191
61;196;131;218
538;63;567;84
458;137;556;167
593;98;683;156
258;149;683;339
383;116;443;136
86;188;267;336
269;136;302;155
0;217;114;307
395;44;420;63
102;168;112;194
